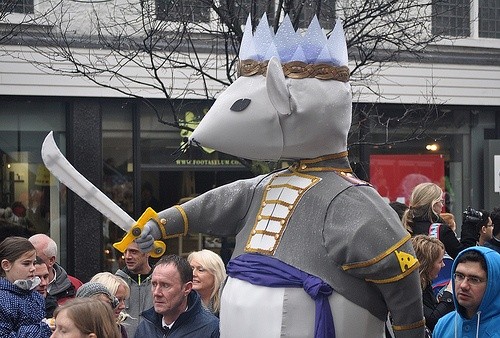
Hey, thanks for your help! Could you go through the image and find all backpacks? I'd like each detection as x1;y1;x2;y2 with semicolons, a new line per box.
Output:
429;222;454;303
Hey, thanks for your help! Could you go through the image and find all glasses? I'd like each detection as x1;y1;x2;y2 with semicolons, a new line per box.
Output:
485;224;494;229
436;200;445;204
453;273;487;284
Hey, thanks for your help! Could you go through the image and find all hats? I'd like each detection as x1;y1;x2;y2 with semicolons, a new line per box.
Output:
76;282;120;309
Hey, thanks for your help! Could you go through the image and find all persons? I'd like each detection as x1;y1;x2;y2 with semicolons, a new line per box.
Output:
490;207;500;248
28;234;77;303
113;232;154;338
49;297;123;338
76;281;120;310
432;247;500;338
385;234;456;338
134;253;221;338
440;213;458;236
474;207;500;253
388;202;409;222
187;249;228;319
90;272;130;338
402;183;483;304
0;237;55;338
35;255;59;319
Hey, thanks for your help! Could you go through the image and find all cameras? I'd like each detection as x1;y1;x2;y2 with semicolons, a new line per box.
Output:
463;209;483;217
